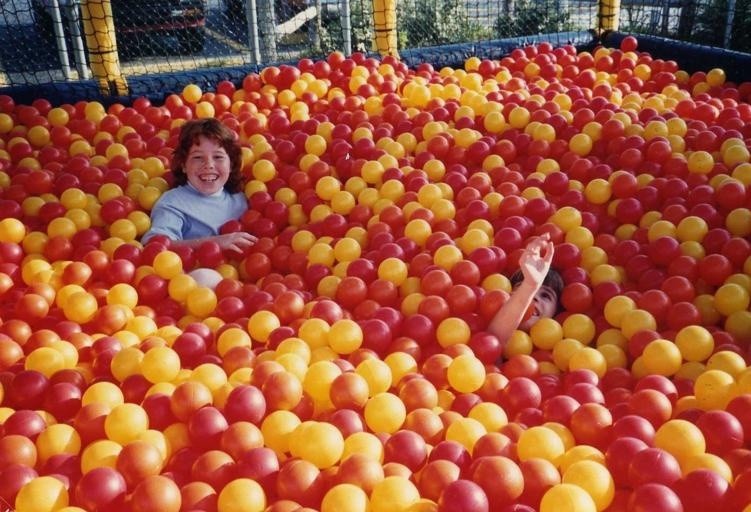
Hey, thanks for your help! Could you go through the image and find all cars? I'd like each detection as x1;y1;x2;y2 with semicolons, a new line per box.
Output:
31;1;207;59
219;1;371;32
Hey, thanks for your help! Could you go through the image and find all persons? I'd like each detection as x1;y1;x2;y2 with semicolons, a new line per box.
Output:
139;117;258;254
483;225;563;366
